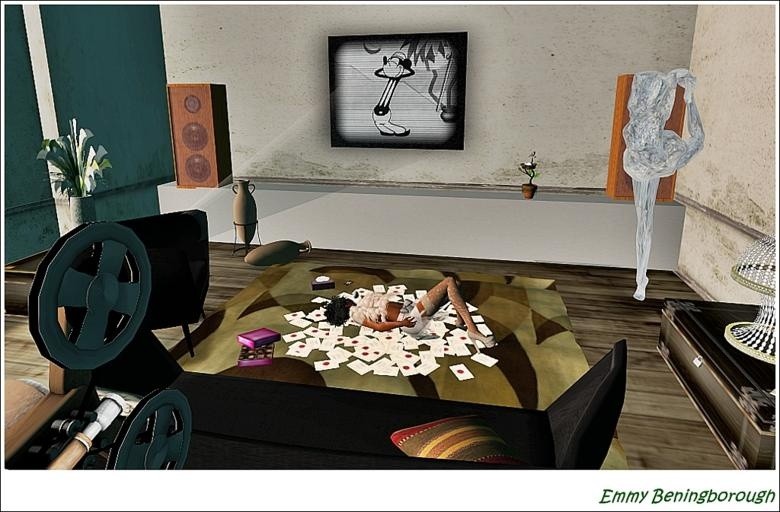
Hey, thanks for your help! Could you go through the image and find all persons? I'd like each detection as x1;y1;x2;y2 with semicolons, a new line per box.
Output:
324;274;494;355
623;67;707;303
